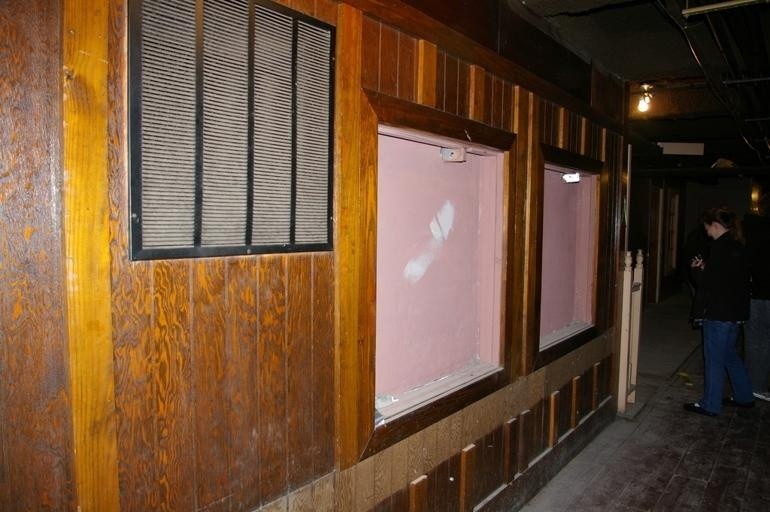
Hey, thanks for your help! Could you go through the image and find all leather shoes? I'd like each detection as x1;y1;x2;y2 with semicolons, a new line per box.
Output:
683;402;717;416
722;397;756;408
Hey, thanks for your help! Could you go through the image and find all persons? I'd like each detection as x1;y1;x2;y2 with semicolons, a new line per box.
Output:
742;193;770;401
683;204;756;418
684;211;710;332
